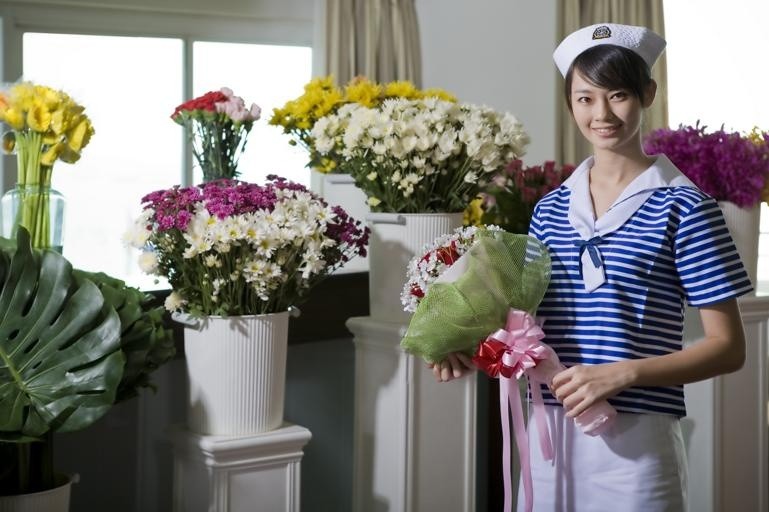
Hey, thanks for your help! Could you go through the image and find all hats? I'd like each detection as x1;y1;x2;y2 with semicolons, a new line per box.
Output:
552;23;666;79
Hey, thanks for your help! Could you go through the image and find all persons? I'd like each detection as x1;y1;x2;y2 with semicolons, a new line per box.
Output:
424;22;755;510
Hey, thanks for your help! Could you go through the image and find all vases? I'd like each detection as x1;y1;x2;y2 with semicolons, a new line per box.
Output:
0;467;72;512
169;308;291;445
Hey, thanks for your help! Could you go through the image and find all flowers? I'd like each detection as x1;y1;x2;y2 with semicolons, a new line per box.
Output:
0;77;769;318
